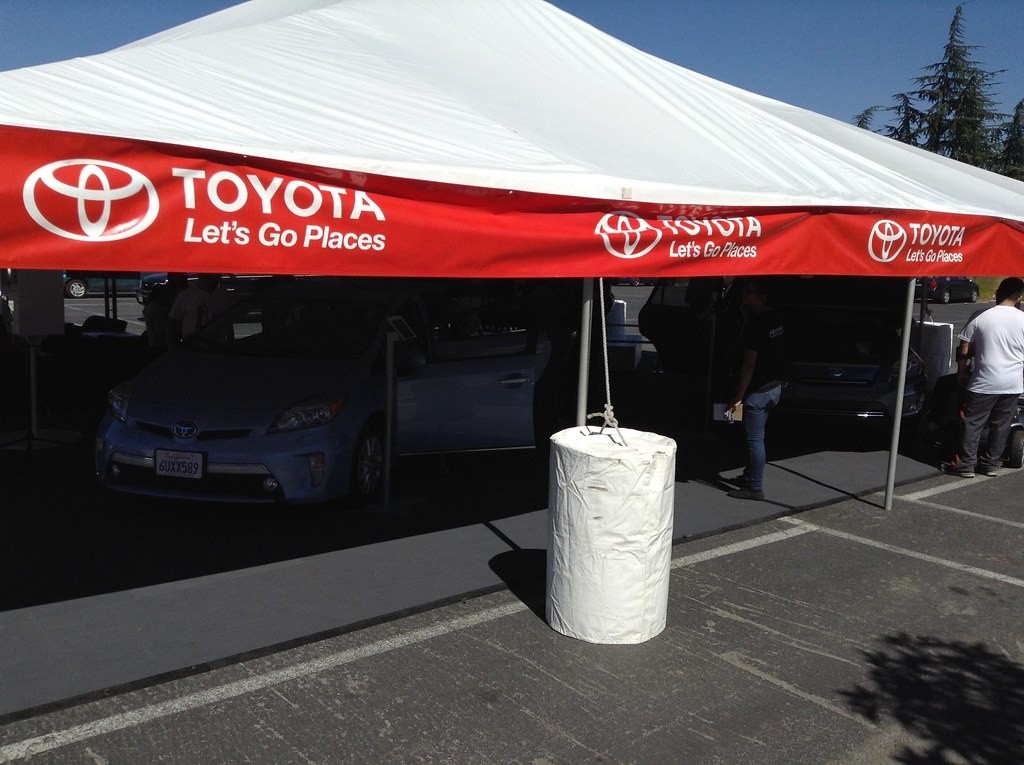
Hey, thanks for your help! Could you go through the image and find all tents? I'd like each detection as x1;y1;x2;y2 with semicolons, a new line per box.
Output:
0;3;1024;512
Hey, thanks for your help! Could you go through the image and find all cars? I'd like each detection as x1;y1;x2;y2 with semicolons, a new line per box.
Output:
64;269;141;298
912;277;980;305
94;275;552;506
136;272;203;304
607;277;659;286
748;275;929;425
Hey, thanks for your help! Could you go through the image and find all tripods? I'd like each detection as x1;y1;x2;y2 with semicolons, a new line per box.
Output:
0;336;61;461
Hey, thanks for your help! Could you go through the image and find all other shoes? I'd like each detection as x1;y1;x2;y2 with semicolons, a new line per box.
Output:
727;486;765;501
729;476;751;487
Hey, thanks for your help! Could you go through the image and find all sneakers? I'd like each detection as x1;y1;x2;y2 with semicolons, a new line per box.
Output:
940;461;975;478
975;458;1000;477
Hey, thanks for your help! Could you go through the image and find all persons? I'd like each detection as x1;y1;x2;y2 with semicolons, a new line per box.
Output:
143;273;192;347
168;275;235;352
940;277;1024;478
724;276;785;501
